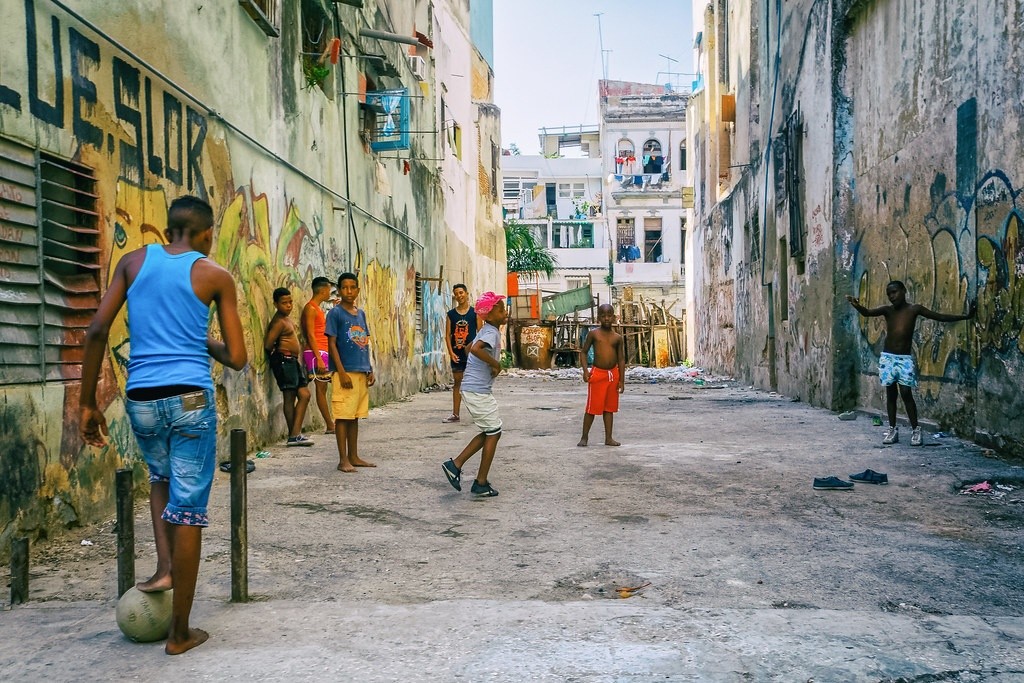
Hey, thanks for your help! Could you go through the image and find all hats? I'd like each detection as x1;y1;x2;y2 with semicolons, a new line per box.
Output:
473;291;506;320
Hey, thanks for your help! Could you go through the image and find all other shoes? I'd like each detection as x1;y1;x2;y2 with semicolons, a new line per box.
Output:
287;434;314;445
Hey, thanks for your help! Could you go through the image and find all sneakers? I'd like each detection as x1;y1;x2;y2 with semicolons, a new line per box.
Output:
872;416;881;425
471;478;499;496
442;414;460;423
910;425;923;445
442;458;462;491
813;476;854;490
848;469;888;485
882;426;899;444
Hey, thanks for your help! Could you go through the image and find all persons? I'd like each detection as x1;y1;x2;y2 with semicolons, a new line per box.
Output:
443;282;484;423
76;193;247;654
301;275;341;434
441;290;510;496
576;303;626;446
843;279;978;446
265;287;316;446
323;272;377;472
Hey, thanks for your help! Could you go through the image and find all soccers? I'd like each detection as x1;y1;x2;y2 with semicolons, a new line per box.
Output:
116;586;175;642
